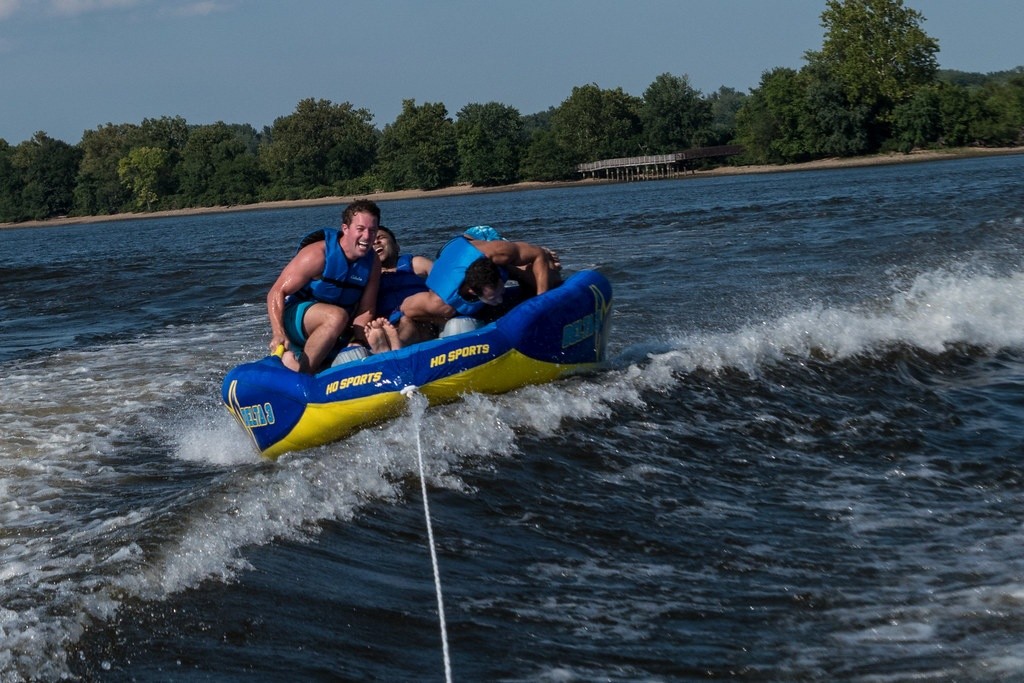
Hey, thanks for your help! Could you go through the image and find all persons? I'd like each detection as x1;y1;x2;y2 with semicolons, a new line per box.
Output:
400;226;562;325
364;226;435;354
266;198;382;374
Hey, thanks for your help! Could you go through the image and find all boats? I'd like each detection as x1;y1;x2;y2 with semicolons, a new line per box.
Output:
220;269;612;465
